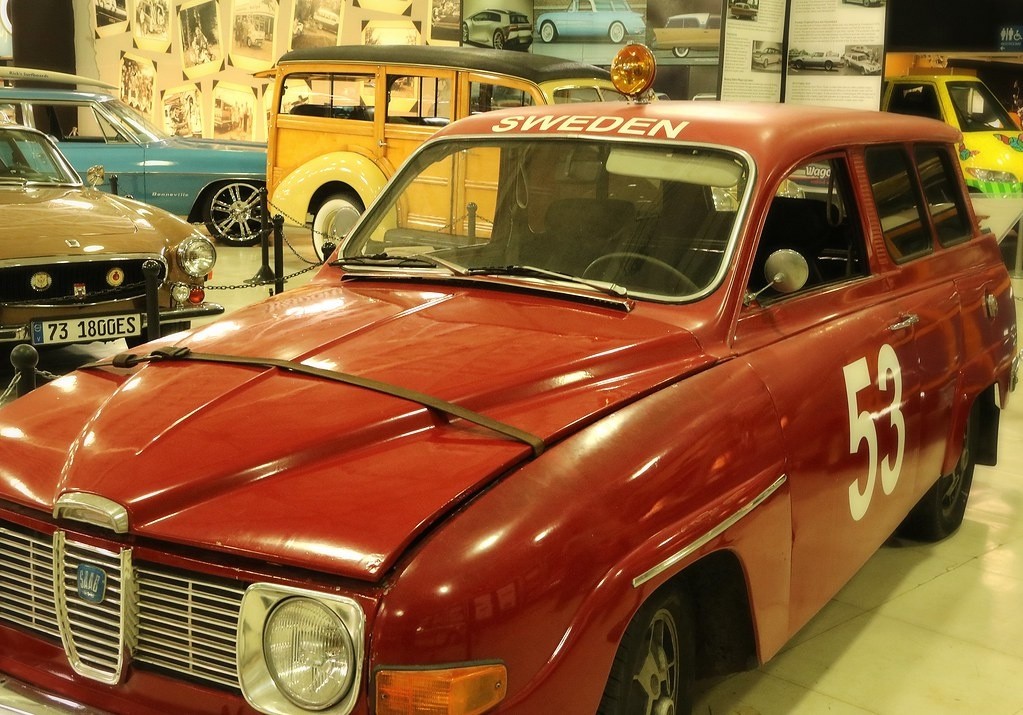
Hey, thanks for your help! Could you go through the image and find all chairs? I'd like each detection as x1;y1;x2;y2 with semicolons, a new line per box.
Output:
748;195;838;301
524;198;634;274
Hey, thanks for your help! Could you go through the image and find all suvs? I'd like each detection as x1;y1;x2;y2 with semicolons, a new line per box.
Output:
462;7;533;51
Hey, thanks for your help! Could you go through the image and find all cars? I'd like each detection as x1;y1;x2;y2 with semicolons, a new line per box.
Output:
535;0;647;45
650;12;721;59
731;3;758;20
842;0;881;7
753;46;782;68
844;52;881;76
790;50;840;71
0;87;274;248
851;45;873;55
0;121;228;350
253;44;661;267
881;74;1023;237
0;43;1023;715
